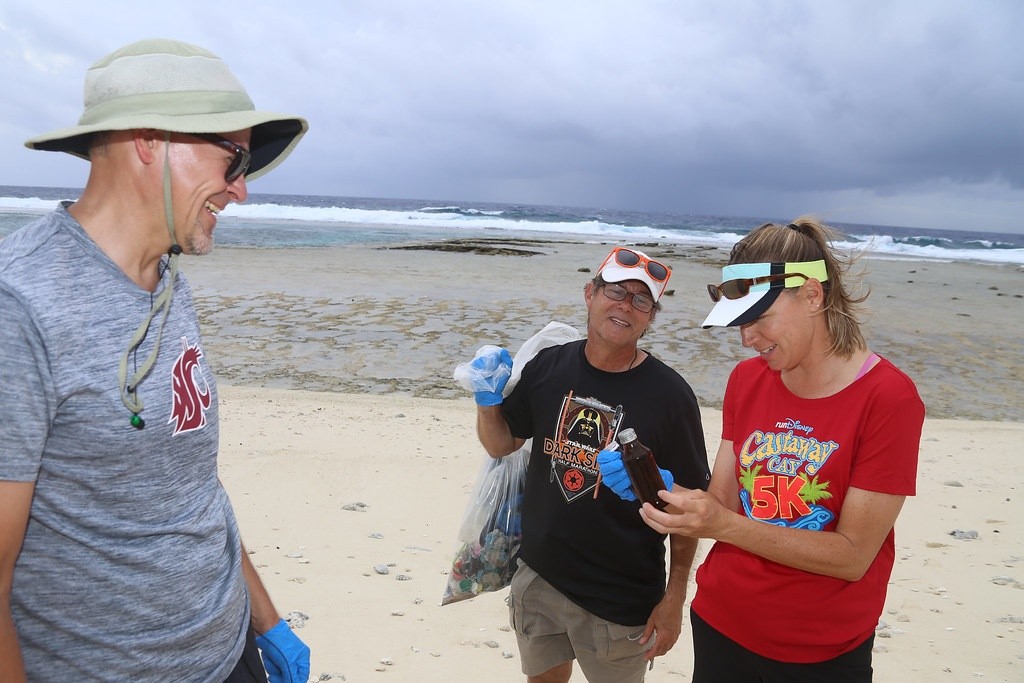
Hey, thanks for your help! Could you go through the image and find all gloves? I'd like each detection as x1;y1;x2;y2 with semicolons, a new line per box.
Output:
471;349;513;406
596;451;674;501
255;618;310;683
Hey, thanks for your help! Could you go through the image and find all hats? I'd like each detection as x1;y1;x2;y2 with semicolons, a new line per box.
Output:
24;40;308;183
701;259;829;329
598;248;668;303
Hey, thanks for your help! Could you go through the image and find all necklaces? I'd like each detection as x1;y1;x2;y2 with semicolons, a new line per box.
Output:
629;350;637;370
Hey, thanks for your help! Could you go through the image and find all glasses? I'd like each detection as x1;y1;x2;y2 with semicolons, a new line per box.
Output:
707;272;810;303
185;130;253;183
594;283;656;313
596;246;672;300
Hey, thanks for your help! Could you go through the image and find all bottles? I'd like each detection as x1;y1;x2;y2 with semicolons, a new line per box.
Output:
618;428;670;510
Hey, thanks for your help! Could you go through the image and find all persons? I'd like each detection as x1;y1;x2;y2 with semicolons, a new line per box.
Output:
470;246;711;683
597;216;925;683
0;41;311;683
